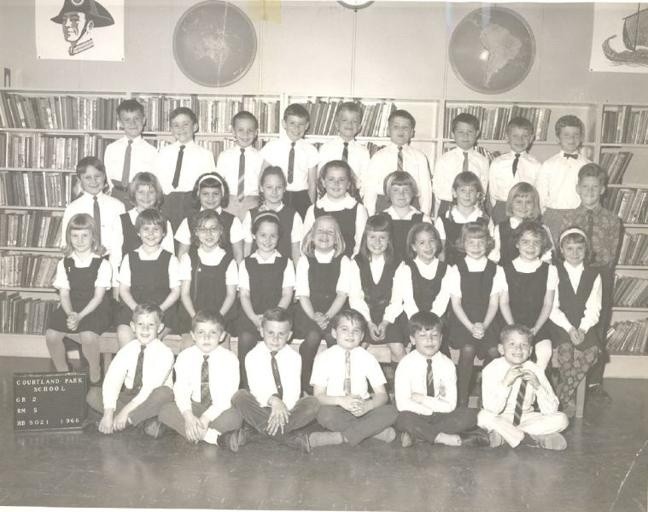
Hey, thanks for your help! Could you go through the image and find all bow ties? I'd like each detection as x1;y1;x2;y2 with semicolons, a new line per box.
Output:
564;153;578;159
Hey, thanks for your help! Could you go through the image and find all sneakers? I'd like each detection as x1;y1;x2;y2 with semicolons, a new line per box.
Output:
460;428;505;448
376;428;395;442
589;384;610;403
218;429;255;452
286;431;344;452
144;417;162;439
402;432;419;447
530;431;567;451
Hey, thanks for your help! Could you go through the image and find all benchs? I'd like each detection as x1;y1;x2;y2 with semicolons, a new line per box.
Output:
99;329;590;421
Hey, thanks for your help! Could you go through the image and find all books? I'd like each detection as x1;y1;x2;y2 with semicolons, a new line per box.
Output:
0;93;648;355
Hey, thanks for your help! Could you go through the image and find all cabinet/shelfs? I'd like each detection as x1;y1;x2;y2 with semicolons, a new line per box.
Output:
0;89;127;336
594;105;648;358
132;94;284;160
440;102;598;173
289;96;438;199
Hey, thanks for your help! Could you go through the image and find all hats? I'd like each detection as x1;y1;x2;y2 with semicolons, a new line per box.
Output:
50;0;114;27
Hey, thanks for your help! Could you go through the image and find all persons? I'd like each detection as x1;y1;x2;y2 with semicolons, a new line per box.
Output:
45;101;623;453
51;0;114;56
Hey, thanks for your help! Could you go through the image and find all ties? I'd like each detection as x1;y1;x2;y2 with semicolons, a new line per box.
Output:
271;351;283;399
93;195;101;249
132;345;145;394
426;359;435;397
238;149;245;202
514;375;527;426
588;209;593;253
172;145;185;187
344;351;351;397
398;146;403;172
287;143;295;184
122;140;133;190
512;153;520;177
463;152;468;170
342;142;349;162
201;355;212;410
